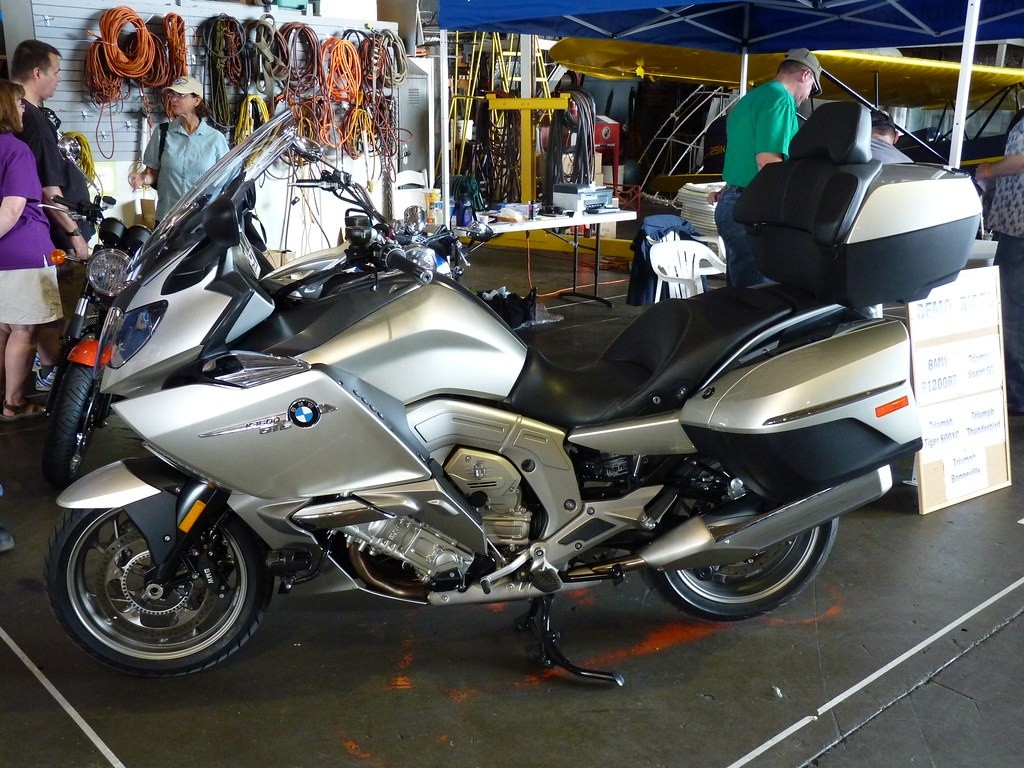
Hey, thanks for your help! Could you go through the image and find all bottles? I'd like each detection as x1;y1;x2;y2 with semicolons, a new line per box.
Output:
434;194;443;225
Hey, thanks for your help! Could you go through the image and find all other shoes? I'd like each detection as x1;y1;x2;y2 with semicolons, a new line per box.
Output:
1008;405;1024;416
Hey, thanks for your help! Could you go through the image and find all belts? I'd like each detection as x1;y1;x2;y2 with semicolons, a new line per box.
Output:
724;182;745;193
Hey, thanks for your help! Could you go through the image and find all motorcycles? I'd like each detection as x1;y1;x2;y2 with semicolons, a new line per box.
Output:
38;196;162;483
46;100;985;689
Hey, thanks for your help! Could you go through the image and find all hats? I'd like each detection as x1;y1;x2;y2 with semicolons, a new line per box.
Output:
776;48;822;98
870;109;895;130
161;76;203;100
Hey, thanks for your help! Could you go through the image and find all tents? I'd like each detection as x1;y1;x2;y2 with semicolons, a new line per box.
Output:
438;0;1024;229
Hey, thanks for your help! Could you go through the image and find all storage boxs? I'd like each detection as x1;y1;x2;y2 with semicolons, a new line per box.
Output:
264;251;295;268
140;199;157;231
562;153;603;186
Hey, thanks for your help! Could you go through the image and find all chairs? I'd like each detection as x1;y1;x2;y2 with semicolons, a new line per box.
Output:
642;215;726;305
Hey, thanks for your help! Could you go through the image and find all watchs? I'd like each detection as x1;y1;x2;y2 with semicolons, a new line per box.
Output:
65;228;82;236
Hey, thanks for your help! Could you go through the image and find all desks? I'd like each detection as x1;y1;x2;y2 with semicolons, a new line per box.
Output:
450;206;636;307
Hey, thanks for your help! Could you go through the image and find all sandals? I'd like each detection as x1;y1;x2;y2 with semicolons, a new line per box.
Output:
0;399;47;421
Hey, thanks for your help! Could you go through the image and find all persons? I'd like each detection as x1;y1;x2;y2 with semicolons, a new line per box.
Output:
0;482;14;552
0;79;64;421
715;48;822;288
131;76;233;235
870;109;915;164
707;184;728;205
974;115;1024;416
10;39;96;392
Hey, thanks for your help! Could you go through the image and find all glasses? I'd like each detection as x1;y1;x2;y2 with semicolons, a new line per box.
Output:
167;93;189;100
38;105;61;130
16;104;25;112
798;70;818;96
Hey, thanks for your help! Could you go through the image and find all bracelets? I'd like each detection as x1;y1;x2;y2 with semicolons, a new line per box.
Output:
976;162;991;177
145;178;146;185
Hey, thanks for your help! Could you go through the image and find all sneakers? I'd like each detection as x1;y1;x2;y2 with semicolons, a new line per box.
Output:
36;368;56;391
33;356;42;369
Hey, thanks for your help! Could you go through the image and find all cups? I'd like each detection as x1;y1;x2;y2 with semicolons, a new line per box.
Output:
480;216;488;226
